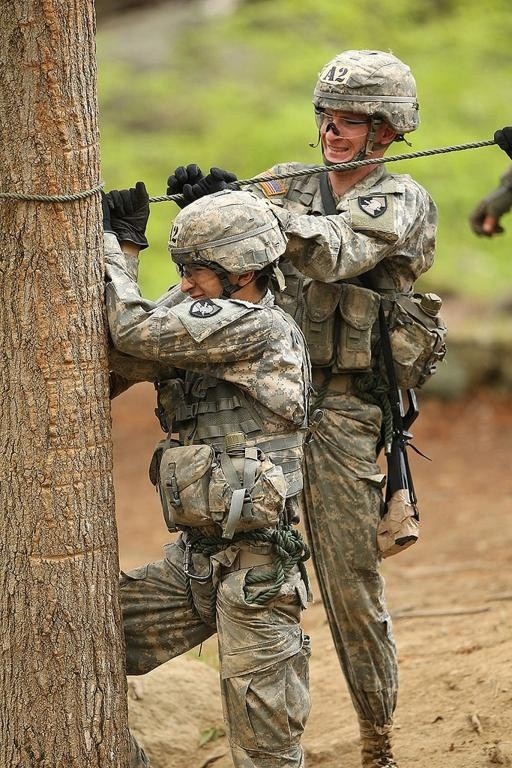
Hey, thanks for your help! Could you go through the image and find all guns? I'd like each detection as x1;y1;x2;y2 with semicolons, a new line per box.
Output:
376;387;419;558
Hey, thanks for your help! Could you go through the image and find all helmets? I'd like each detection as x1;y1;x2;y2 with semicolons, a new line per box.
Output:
167;189;290;276
309;47;421;135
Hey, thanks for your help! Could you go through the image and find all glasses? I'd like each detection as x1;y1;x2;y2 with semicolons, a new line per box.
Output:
312;106;378;139
171;257;228;284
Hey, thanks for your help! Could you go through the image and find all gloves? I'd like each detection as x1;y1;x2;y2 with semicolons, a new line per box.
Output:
165;161;243;210
94;178;153;250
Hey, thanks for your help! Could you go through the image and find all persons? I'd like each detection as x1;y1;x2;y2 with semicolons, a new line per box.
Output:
468;167;511;238
493;124;511;160
110;47;447;768
98;180;323;768
